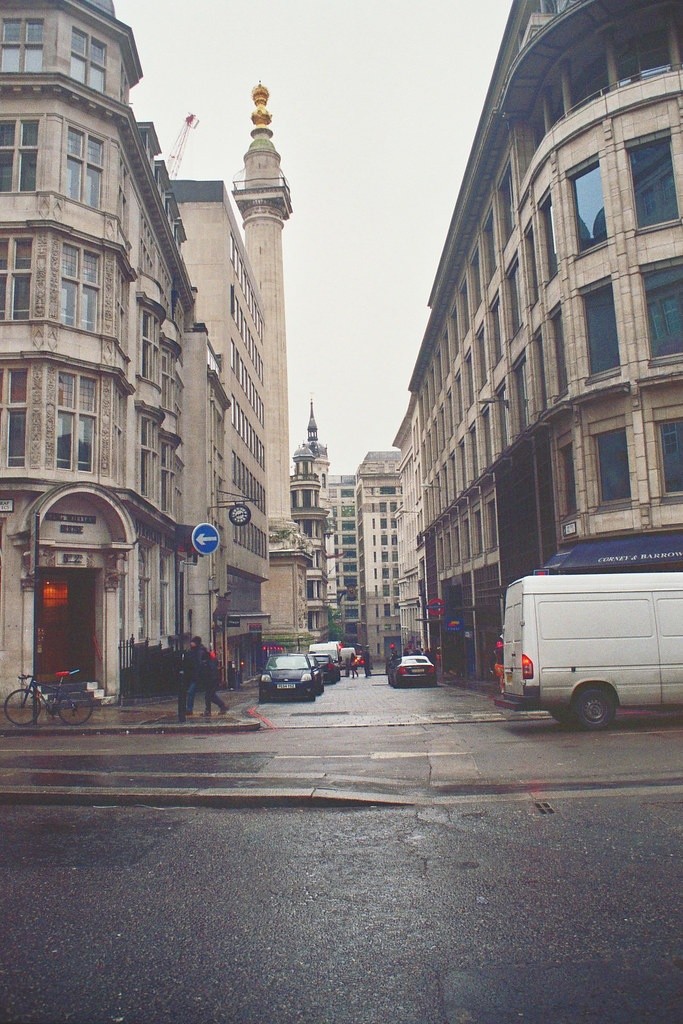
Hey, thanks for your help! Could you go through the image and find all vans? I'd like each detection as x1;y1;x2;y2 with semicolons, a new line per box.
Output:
494;572;683;732
308;640;365;671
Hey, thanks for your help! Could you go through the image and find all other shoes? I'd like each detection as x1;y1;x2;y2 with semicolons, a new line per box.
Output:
185;710;193;714
200;712;211;717
218;705;230;715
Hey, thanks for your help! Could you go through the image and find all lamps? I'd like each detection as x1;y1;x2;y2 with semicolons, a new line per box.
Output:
420;483;442;492
399;510;422;517
477;397;509;410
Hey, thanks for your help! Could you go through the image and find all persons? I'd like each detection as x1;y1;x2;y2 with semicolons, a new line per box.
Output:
388;647;441;671
350;652;359;678
361;649;371;677
185;636;229;718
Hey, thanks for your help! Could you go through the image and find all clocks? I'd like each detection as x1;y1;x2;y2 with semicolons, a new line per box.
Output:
229;504;250;526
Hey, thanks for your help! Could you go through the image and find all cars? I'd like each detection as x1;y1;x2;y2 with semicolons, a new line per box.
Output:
310;653;341;684
306;653;325;695
387;655;438;689
257;654;317;702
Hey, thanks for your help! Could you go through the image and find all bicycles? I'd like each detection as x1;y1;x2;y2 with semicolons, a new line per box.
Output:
3;671;93;726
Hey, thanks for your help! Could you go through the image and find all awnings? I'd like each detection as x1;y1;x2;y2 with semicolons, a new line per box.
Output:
543;531;683;569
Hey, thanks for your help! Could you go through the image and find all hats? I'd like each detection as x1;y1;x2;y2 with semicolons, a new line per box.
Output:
190;636;201;643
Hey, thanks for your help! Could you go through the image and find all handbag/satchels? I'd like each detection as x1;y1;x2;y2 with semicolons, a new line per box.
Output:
352;659;359;667
208;652;219;670
